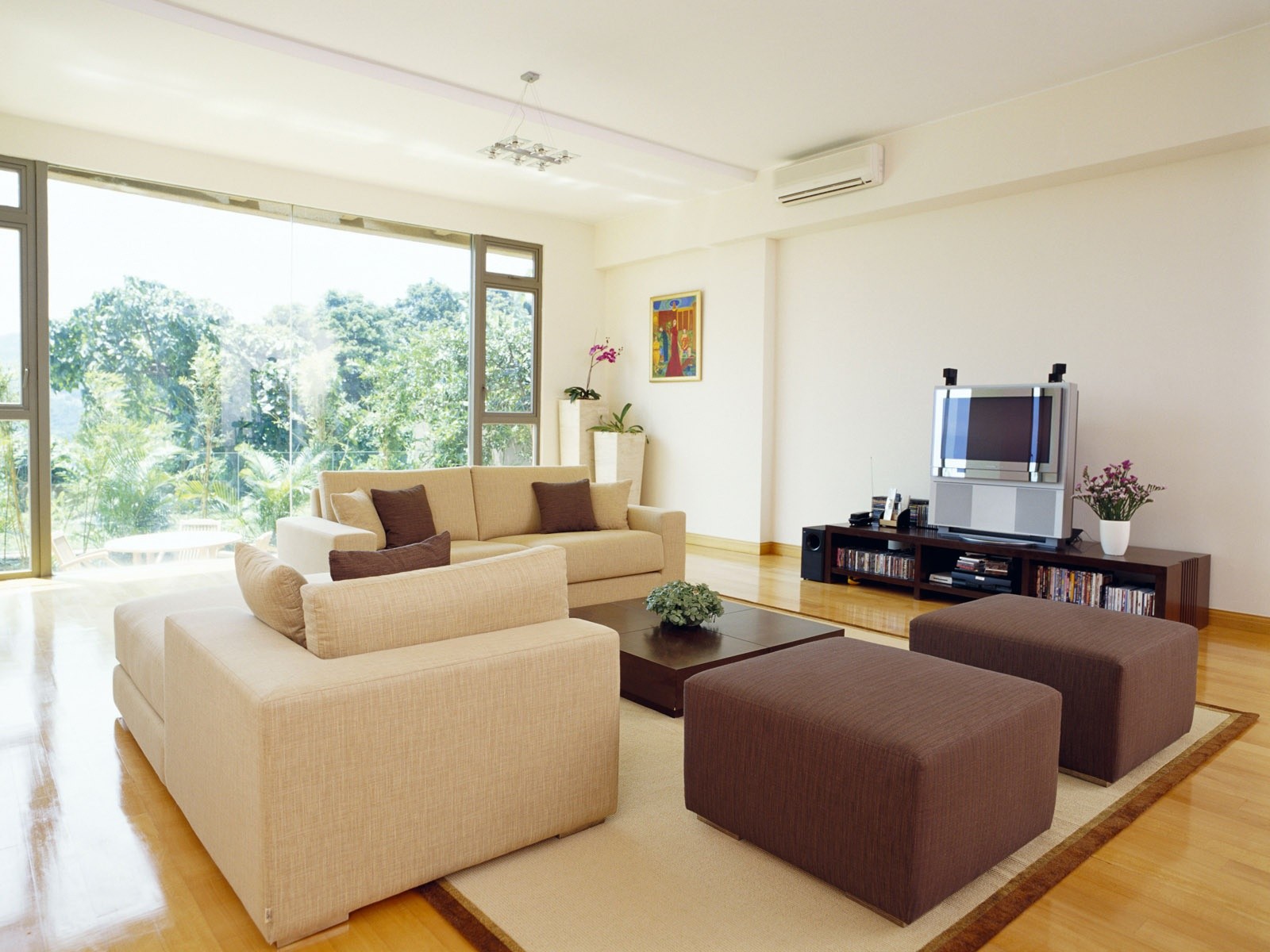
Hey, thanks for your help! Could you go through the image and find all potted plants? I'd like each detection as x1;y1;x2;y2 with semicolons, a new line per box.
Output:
594;406;650;505
646;582;725;633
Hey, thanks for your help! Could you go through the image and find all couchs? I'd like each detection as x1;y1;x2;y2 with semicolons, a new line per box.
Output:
110;539;623;945
277;465;686;611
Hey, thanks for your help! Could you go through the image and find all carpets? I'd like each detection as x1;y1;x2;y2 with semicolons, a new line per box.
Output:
417;685;1260;952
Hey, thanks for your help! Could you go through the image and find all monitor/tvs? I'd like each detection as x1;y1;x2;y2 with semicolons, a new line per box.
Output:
931;387;1065;483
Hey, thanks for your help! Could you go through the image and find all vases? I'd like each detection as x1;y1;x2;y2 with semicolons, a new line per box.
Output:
561;398;608;479
1097;516;1132;555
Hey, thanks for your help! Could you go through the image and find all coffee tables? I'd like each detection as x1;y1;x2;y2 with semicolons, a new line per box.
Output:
572;594;846;717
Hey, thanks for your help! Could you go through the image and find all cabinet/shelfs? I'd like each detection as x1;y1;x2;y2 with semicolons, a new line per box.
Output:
800;529;1211;631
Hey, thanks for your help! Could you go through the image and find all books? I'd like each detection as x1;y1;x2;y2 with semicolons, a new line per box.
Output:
1036;565;1155;616
954;557;1009;575
835;547;917;581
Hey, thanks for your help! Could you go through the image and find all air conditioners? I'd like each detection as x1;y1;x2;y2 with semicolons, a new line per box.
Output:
774;144;886;205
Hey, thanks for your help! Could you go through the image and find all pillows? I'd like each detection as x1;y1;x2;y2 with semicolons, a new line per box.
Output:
325;531;454;585
532;476;590;533
328;489;394;548
300;546;568;655
234;544;306;645
590;479;634;528
370;485;436;547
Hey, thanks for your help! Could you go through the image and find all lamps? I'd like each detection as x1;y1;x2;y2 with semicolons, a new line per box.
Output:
477;70;580;173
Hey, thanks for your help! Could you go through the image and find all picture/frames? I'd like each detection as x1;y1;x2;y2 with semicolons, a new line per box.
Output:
648;292;701;382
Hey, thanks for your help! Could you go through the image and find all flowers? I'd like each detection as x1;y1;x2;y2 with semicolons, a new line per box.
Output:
564;341;627;402
1070;460;1164;520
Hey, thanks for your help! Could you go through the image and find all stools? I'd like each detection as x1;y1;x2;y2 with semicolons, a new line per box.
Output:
682;642;1064;927
910;594;1201;783
